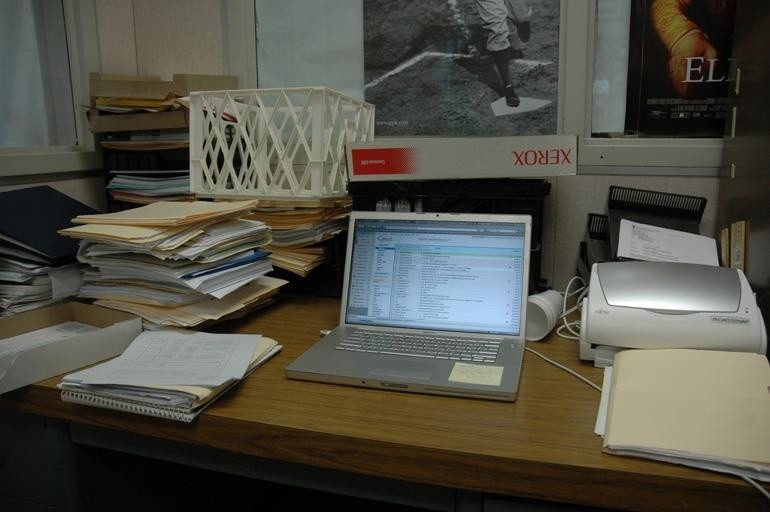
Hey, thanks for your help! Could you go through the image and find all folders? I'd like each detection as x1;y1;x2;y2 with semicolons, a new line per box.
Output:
0;185;106;268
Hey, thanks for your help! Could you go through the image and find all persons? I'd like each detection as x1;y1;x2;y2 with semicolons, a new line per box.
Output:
650;1;717;97
477;0;531;107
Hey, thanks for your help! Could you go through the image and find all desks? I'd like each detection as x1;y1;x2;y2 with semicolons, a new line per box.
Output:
0;296;770;512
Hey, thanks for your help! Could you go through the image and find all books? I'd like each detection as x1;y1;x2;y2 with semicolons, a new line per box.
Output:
602;346;770;485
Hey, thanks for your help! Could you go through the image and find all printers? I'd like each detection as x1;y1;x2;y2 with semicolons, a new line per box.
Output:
578;261;770;369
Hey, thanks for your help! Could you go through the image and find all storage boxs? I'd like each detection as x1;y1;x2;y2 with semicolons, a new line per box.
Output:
0;302;143;394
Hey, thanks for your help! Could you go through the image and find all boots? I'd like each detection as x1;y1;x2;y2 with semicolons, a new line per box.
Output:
516;20;531;44
490;46;522;108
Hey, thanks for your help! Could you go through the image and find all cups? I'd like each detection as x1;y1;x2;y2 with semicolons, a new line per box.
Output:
524;287;564;346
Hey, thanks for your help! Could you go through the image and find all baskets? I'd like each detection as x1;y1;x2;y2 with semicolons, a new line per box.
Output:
188;86;380;204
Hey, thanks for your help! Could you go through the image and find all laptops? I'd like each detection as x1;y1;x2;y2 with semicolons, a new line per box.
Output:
285;208;534;403
